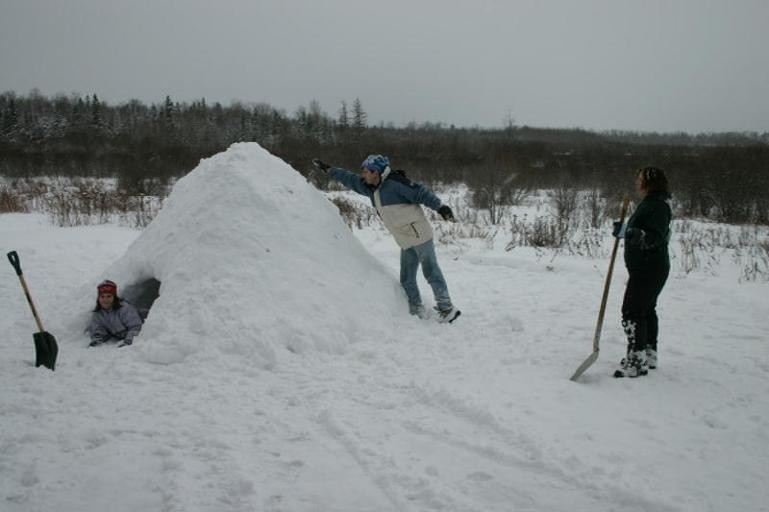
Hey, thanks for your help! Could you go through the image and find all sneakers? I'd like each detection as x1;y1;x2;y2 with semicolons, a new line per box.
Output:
615;344;656;377
433;305;461;323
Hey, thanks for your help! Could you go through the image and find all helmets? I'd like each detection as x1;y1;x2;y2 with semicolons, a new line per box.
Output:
361;154;390;174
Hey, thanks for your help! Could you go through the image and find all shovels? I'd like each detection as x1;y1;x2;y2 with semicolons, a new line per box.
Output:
7;250;58;371
569;194;631;381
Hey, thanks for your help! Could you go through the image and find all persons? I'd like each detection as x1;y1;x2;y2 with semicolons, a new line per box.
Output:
88;281;150;350
613;166;671;378
311;154;461;324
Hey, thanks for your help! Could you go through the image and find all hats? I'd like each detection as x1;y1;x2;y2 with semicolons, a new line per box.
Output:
98;280;117;294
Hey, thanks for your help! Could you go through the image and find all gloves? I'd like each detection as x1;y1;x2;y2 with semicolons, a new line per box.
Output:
118;339;131;347
612;222;627;238
89;340;103;346
437;205;454;221
312;158;331;173
